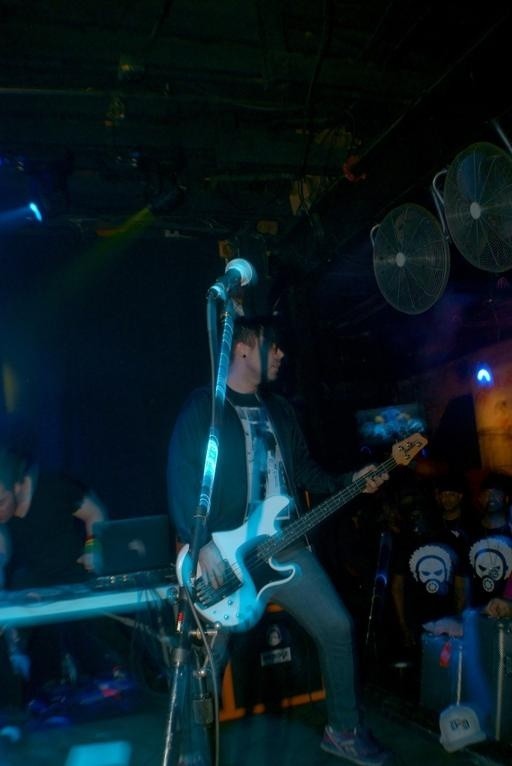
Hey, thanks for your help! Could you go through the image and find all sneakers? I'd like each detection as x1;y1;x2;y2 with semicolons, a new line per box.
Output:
319;724;389;766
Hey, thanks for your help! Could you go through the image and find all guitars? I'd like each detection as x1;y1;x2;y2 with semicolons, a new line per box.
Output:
175;433;428;632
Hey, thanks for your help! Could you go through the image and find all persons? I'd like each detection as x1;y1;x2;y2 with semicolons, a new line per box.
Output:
165;322;393;765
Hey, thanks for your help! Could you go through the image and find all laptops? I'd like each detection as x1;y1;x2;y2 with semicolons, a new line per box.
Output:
91;514;172;578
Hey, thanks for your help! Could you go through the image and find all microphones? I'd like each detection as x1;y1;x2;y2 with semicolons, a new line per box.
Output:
205;257;256;300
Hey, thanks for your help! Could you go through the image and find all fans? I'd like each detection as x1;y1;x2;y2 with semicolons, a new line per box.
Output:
432;137;511;276
369;199;451;317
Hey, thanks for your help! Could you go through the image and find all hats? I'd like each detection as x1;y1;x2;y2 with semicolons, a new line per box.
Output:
438;705;486;754
